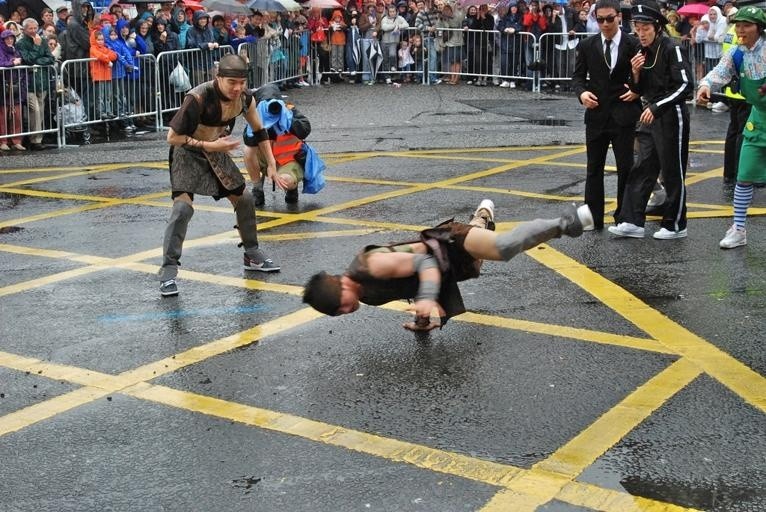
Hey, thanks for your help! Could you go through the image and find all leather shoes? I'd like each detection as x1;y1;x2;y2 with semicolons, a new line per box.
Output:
279;84;286;90
285;83;294;89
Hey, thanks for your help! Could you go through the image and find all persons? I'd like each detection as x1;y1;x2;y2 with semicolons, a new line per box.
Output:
694;5;766;249
293;0;590;90
664;0;738;112
586;0;636;39
242;98;311;205
0;1;293;152
572;0;641;231
722;22;752;183
156;54;280;296
607;5;694;239
303;200;594;331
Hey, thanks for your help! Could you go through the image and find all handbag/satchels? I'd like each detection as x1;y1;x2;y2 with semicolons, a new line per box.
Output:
423;9;442;52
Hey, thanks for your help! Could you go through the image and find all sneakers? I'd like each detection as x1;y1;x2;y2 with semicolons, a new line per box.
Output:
119;125;131;131
510;81;515;89
297;81;310;87
242;253;280;271
368;80;375;86
653;224;690;238
414;78;423;85
719;226;747;247
286;190;298;202
30;143;44;149
475;79;482;86
0;143;9;151
160;279;177;296
385;77;391;83
482;79;488;86
401;76;412;83
433;77;442;85
500;81;509;88
475;198;495;220
378;78;383;83
13;144;26;150
347;77;356;85
466;79;473;84
127;125;135;131
607;223;646;237
572;203;595;233
251;189;264;204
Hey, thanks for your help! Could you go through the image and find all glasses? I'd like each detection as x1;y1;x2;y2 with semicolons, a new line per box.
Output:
595;15;619;22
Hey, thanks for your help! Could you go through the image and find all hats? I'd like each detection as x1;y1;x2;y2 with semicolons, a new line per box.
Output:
730;8;766;27
632;6;667;25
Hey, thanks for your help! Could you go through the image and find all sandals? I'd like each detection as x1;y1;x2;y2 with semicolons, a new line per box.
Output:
448;78;459;85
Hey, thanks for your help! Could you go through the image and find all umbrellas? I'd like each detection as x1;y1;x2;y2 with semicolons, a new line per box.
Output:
198;0;253;15
0;0;52;24
117;0;174;4
301;0;342;8
174;0;202;10
675;3;710;16
248;0;287;14
274;0;302;12
